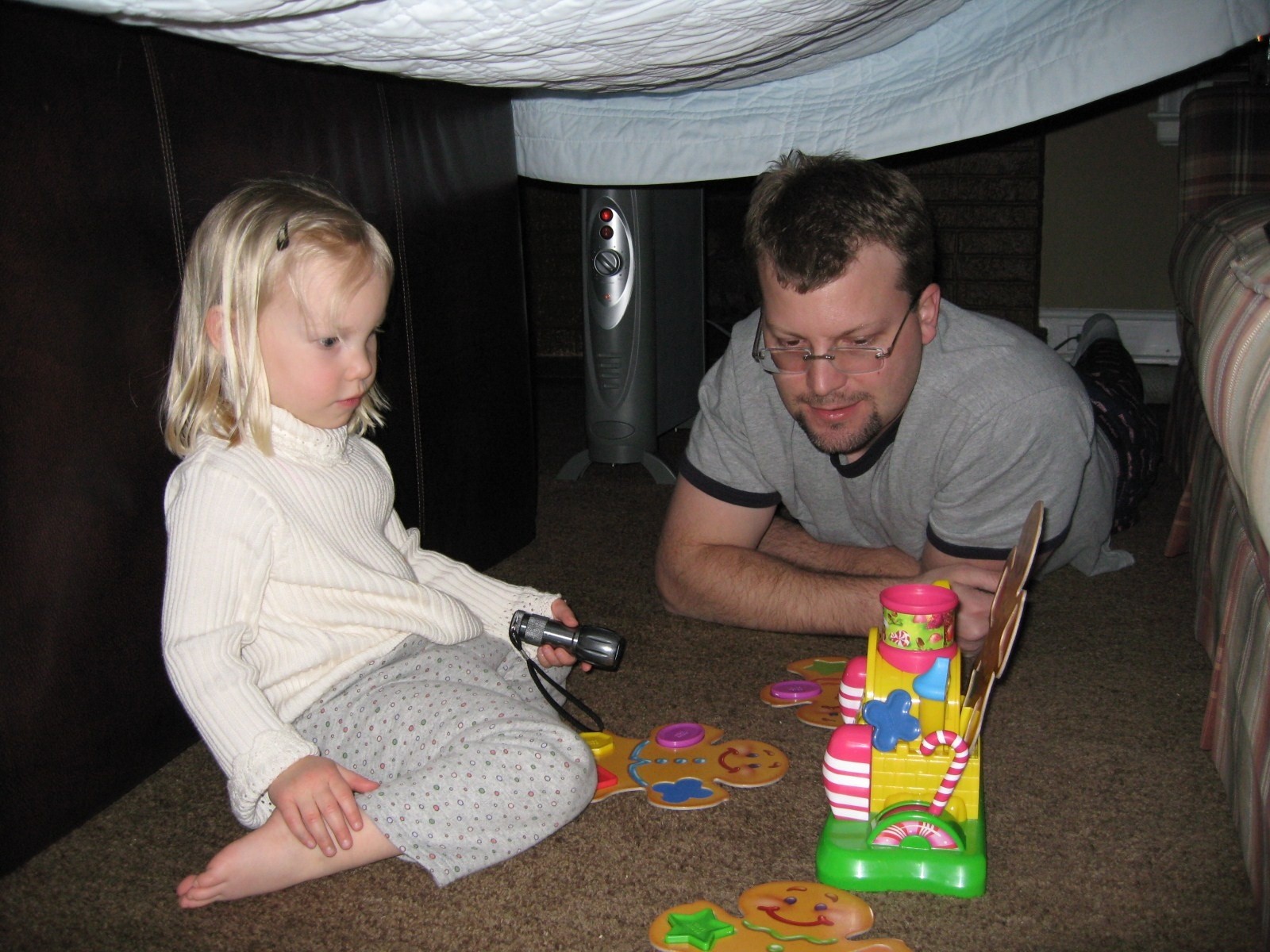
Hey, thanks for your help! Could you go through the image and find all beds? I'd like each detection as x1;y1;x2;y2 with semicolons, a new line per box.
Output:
1165;81;1270;952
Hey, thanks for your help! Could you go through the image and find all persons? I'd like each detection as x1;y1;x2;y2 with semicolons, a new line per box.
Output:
653;149;1148;663
157;176;593;912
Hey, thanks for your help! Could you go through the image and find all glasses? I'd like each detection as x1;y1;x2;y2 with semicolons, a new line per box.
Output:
751;277;920;376
